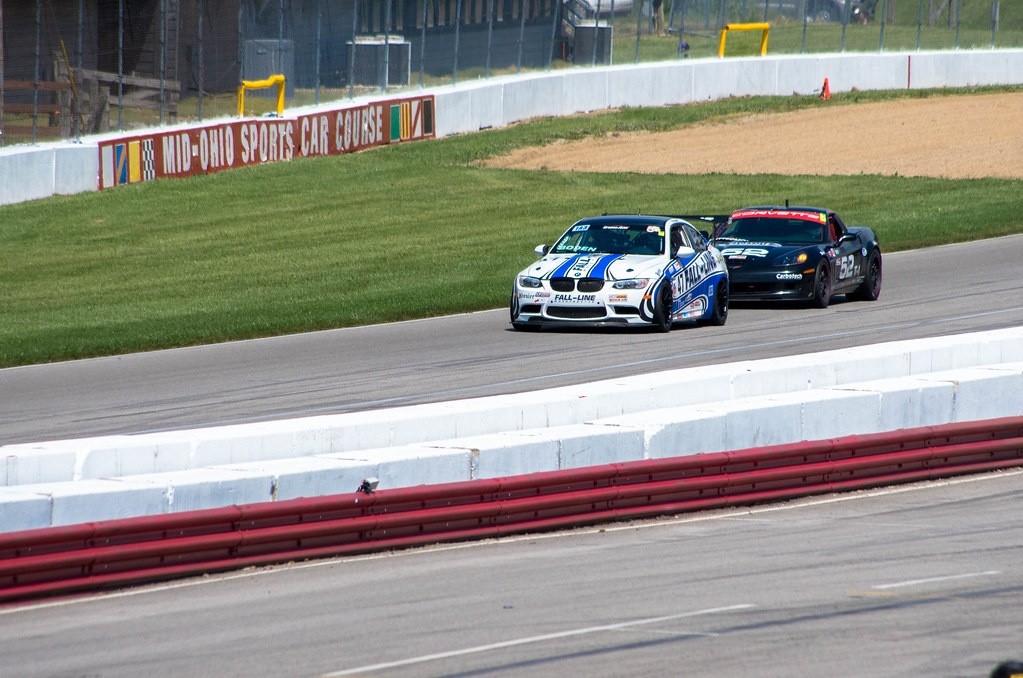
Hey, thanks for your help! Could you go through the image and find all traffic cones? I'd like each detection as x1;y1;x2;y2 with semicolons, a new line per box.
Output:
819;78;831;102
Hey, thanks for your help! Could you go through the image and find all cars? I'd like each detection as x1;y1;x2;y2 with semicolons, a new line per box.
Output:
735;0;880;26
558;0;633;27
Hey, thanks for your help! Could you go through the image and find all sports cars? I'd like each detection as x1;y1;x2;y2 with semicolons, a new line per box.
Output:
698;206;883;308
510;212;732;332
